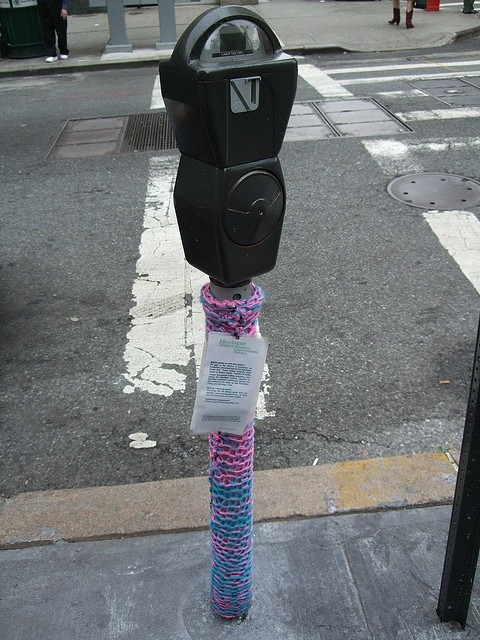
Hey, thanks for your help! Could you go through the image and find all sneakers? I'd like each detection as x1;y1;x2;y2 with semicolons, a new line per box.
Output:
60;54;68;60
46;56;58;63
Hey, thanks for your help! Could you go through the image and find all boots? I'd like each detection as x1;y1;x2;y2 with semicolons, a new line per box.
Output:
388;8;400;25
406;10;414;29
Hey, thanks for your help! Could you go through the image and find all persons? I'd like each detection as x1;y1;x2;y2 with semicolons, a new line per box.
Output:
36;0;69;62
388;0;415;28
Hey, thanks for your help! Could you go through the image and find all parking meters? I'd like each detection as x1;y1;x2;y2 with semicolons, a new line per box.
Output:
159;7;296;621
